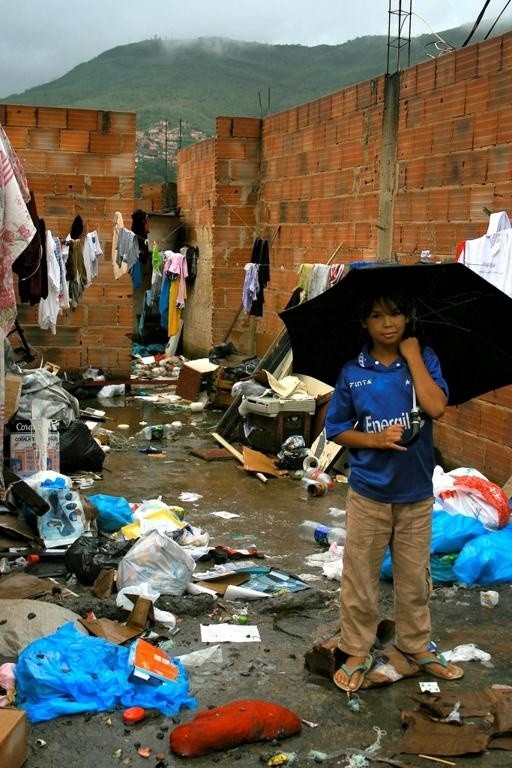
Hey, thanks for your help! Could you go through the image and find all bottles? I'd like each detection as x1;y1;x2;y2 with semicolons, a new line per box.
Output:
299;466;336;498
297;519;347;549
134;422;178;441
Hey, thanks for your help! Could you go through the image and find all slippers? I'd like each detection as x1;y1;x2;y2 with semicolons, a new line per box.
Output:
404;649;465;680
332;653;374;694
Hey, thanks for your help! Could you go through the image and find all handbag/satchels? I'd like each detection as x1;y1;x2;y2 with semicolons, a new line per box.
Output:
464;211;512;300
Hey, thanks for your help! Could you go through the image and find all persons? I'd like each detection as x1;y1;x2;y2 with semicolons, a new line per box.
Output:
321;290;467;694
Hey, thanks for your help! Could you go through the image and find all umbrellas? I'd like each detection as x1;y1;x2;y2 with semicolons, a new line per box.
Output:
278;260;512;407
